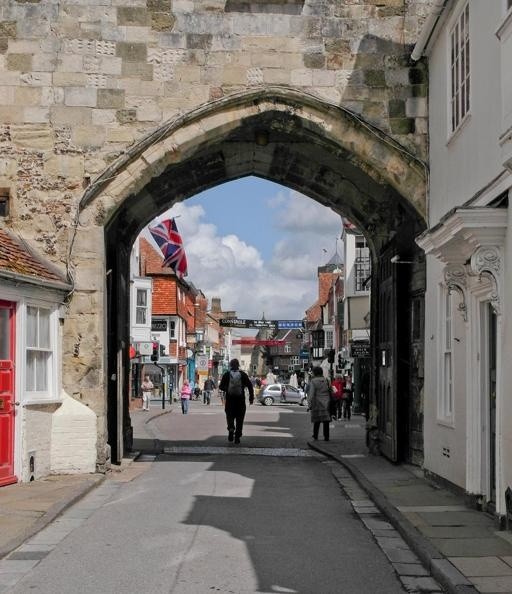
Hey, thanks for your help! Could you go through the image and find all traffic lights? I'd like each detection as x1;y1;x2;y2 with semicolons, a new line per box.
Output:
326;347;335;365
151;343;158;361
159;344;166;357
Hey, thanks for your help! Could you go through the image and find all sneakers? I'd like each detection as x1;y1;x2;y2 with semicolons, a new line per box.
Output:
228;429;234;441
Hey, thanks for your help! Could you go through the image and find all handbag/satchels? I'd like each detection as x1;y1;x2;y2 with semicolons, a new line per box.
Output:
329;398;340;415
342;392;349;399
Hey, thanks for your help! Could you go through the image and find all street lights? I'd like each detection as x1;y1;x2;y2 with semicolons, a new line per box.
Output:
183;302;200;325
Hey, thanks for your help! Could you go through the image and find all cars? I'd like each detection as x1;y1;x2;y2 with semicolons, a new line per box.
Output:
257;384;307;405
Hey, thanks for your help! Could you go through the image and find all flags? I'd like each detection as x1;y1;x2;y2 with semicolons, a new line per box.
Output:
149;218;188;280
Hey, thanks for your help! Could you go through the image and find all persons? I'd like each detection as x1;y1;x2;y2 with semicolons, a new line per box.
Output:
141;376;154;411
180;358;357;444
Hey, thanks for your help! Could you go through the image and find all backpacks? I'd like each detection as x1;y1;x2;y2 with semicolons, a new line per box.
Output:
228;370;243;396
